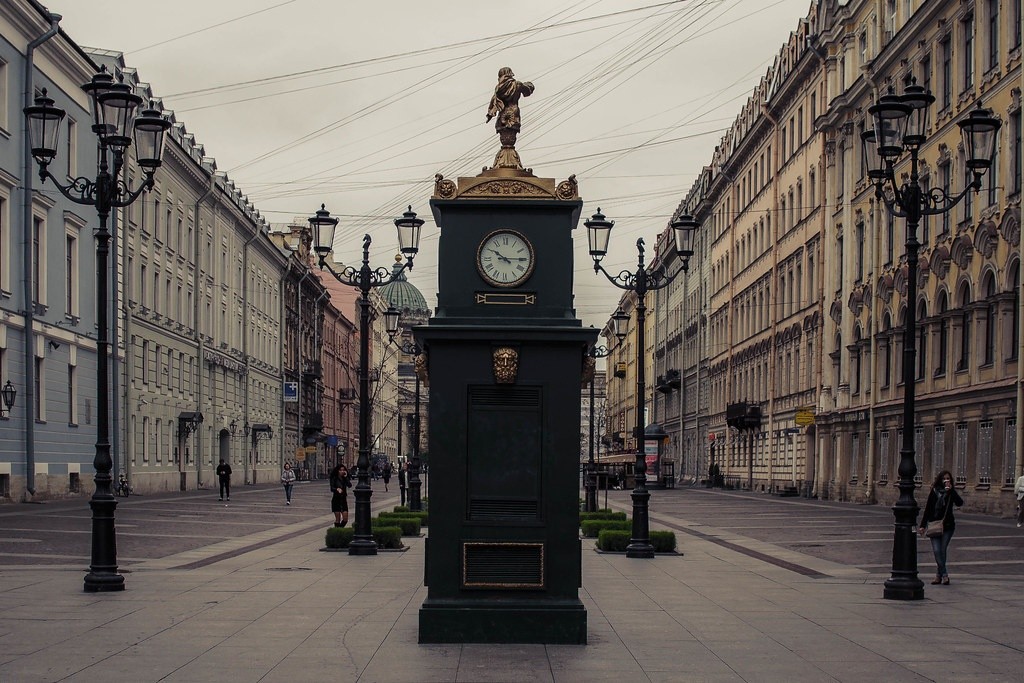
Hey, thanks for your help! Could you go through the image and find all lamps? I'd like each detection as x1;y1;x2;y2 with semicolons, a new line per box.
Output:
49;339;60;350
0;379;17;412
238;422;251;437
217;419;237;441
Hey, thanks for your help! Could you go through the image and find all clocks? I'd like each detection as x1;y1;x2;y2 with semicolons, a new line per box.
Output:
476;227;534;288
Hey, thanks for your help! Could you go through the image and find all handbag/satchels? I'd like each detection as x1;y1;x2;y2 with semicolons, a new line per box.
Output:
925;520;943;538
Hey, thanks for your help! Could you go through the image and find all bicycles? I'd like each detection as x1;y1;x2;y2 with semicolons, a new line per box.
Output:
113;474;130;497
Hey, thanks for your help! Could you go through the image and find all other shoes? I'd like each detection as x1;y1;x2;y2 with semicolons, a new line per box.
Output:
287;501;290;505
931;576;942;585
227;498;230;501
219;498;223;501
1017;523;1023;528
943;577;950;585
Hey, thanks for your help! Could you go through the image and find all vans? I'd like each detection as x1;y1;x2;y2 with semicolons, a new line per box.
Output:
370;453;390;477
397;456;408;471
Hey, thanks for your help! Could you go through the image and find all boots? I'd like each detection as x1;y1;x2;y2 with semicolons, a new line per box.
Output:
340;520;347;528
335;522;340;527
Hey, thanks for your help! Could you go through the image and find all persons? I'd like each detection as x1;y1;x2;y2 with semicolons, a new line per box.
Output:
281;463;296;505
329;464;352;527
373;464;379;481
919;470;963;585
217;458;232;501
398;463;410;506
381;465;390;492
486;65;535;147
1014;476;1024;527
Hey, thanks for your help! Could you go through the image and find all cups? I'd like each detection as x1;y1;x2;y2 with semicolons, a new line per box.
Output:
944;480;950;490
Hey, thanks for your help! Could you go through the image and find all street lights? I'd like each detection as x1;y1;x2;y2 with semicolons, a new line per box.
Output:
23;63;171;590
583;205;697;557
307;202;424;556
859;77;1005;602
587;306;632;513
381;302;424;511
